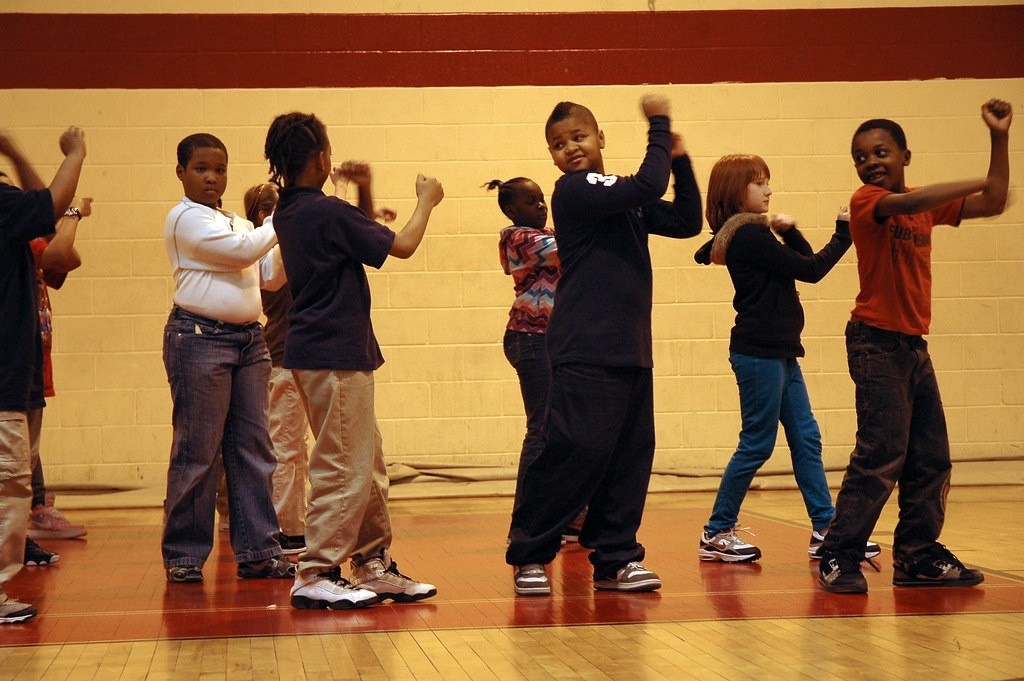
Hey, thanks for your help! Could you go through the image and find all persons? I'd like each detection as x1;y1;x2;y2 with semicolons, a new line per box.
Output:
815;99;1013;592
506;89;703;596
0;123;93;627
216;456;229;534
263;109;445;611
480;179;587;547
245;159;376;554
161;133;298;581
695;153;880;562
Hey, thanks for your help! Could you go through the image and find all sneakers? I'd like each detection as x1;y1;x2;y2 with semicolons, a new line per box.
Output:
236;555;300;579
891;543;985;587
699;523;762;563
593;560;662;592
24;537;59;566
817;546;880;594
25;505;88;539
288;567;378;609
512;561;551;595
278;531;307;555
349;547;437;603
808;531;881;560
0;594;37;623
165;561;204;582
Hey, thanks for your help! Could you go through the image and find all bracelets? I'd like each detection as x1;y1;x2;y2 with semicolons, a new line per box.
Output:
64;207;82;220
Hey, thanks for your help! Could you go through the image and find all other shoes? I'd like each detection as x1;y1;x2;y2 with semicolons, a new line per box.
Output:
506;527;567;547
218;513;230;532
560;526;580;543
